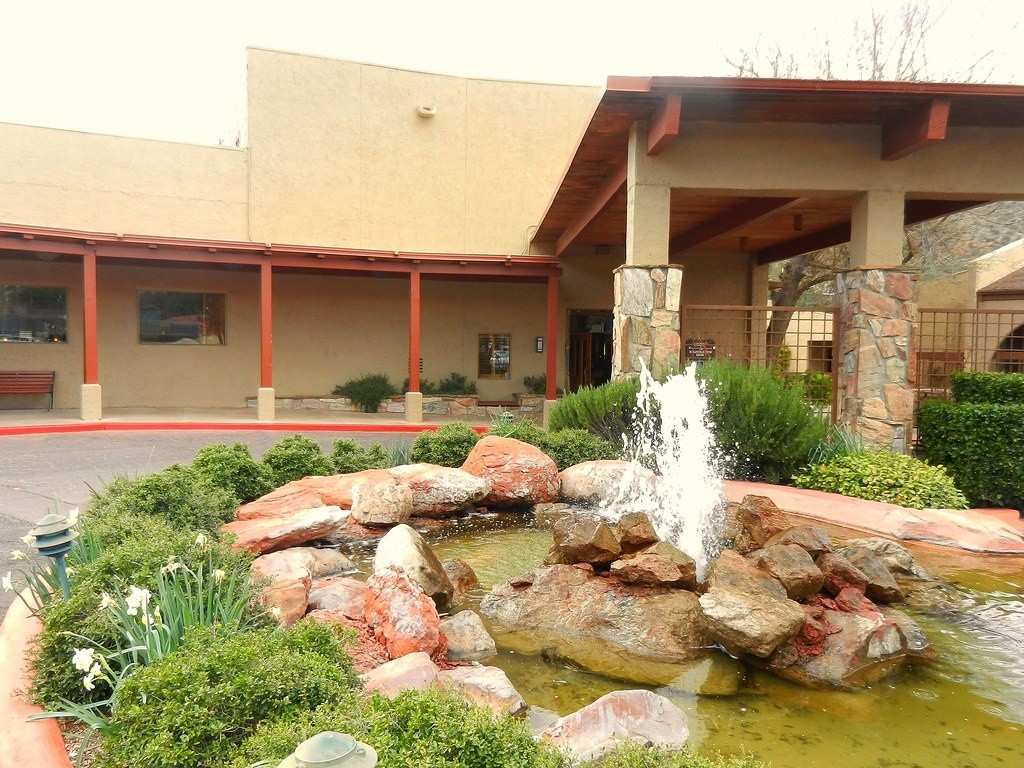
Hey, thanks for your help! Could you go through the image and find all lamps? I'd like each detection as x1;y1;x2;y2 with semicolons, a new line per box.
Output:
536;336;543;352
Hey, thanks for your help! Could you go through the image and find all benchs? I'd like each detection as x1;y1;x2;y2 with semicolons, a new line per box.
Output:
0;371;55;411
